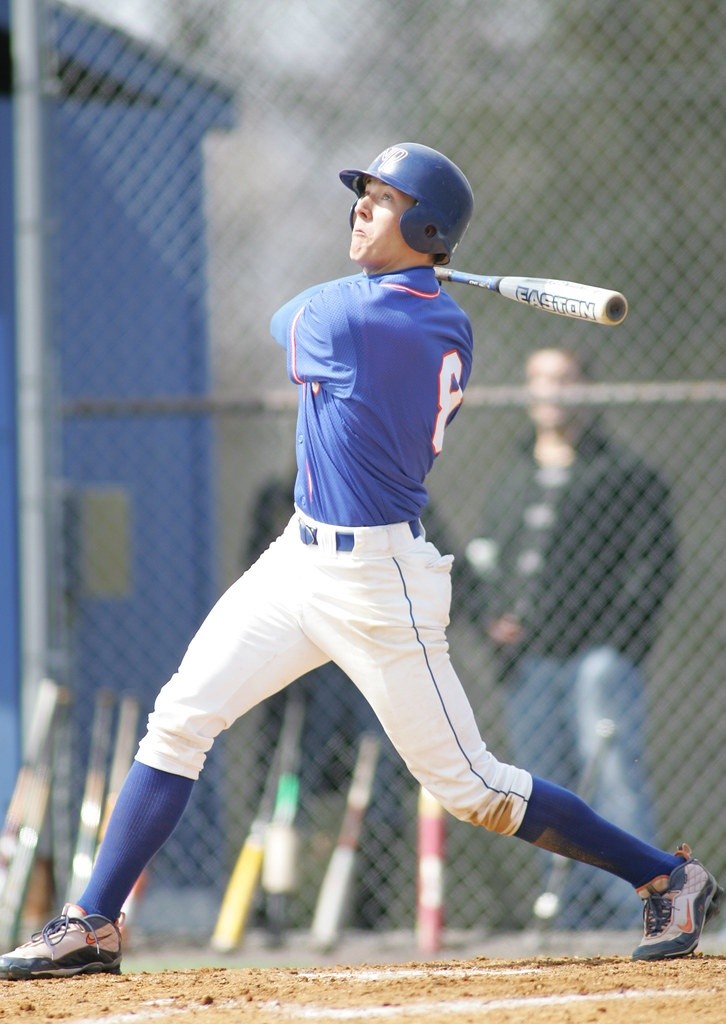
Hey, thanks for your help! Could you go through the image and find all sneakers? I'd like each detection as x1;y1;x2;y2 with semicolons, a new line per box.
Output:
633;843;725;961
0;899;127;980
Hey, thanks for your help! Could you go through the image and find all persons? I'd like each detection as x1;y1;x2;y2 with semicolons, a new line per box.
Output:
242;457;527;928
464;341;682;931
0;141;723;985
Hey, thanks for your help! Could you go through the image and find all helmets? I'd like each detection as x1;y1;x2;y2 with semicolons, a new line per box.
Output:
339;142;473;265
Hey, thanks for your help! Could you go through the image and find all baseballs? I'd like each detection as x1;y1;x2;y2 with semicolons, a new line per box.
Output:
532;891;563;921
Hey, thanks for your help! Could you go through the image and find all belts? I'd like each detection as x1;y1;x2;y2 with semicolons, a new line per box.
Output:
300;521;420;551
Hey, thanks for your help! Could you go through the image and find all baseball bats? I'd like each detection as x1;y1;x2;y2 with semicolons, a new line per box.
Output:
431;263;628;328
